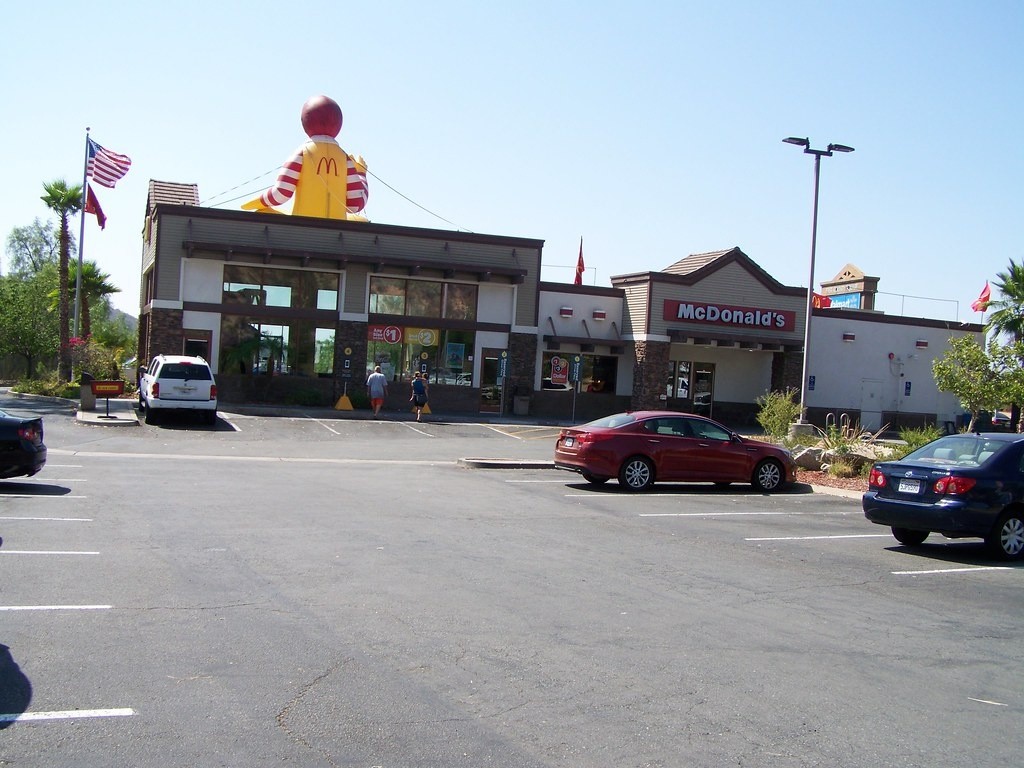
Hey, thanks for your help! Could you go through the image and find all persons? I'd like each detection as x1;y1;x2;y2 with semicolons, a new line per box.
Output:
409;370;429;422
366;366;389;418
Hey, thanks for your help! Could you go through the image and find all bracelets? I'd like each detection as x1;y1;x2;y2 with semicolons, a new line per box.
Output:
368;392;370;393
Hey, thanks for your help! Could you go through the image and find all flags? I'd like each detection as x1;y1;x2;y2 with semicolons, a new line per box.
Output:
83;183;107;230
86;136;132;188
576;244;584;284
971;283;991;312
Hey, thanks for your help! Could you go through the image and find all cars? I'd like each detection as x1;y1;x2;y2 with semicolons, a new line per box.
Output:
554;410;798;491
981;408;1011;425
542;377;575;391
862;433;1024;561
123;357;137;386
666;375;711;405
0;409;47;479
456;373;472;387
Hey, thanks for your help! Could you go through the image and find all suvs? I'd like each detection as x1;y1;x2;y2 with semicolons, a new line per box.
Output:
138;354;217;425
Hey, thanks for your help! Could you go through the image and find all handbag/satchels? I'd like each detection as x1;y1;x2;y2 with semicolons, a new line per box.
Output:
417;394;428;402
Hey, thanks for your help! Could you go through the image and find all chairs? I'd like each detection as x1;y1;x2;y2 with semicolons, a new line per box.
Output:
934;447;956;462
959;453;978;462
978;450;995;466
672;422;704;437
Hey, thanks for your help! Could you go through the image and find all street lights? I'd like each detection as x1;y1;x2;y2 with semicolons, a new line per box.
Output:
782;136;855;445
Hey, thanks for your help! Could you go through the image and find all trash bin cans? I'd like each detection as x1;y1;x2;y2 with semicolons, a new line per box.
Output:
513;387;530;415
79;372;95;410
